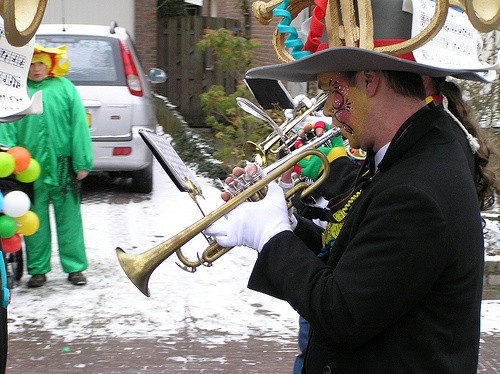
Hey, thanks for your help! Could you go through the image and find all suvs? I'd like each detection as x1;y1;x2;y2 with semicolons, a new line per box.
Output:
32;21;167;193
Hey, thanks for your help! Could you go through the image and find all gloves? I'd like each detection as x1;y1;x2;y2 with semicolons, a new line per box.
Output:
199;175;295;252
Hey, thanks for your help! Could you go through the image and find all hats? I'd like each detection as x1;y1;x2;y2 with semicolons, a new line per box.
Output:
246;0;500;87
31;42;70;77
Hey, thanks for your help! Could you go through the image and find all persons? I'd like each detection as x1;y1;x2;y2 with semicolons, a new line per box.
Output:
0;44;93;288
203;0;500;374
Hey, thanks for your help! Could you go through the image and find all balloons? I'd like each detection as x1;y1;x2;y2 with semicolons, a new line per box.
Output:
0;146;41;253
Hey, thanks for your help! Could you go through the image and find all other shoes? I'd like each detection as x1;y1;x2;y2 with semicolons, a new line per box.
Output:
68;273;87;285
27;274;50;288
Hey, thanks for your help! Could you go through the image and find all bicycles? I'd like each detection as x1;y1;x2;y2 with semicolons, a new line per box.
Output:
0;145;24;281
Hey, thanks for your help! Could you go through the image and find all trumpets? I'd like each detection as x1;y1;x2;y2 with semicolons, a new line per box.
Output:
114;126;344;298
242;91;333;166
